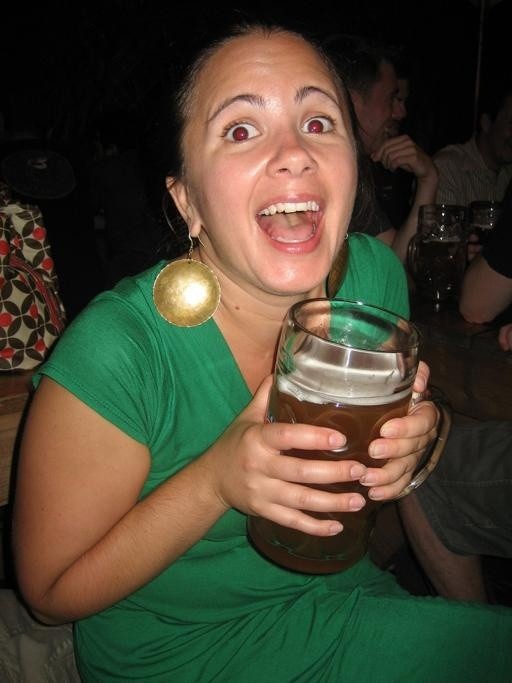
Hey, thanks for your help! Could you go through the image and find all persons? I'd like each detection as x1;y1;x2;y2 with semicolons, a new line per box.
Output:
2;186;69;516
326;25;511;358
9;0;510;683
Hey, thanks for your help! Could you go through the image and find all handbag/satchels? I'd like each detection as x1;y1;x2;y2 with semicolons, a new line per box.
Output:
1;184;70;374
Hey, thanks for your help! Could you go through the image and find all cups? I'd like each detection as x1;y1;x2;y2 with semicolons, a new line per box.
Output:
244;295;451;577
404;198;503;326
